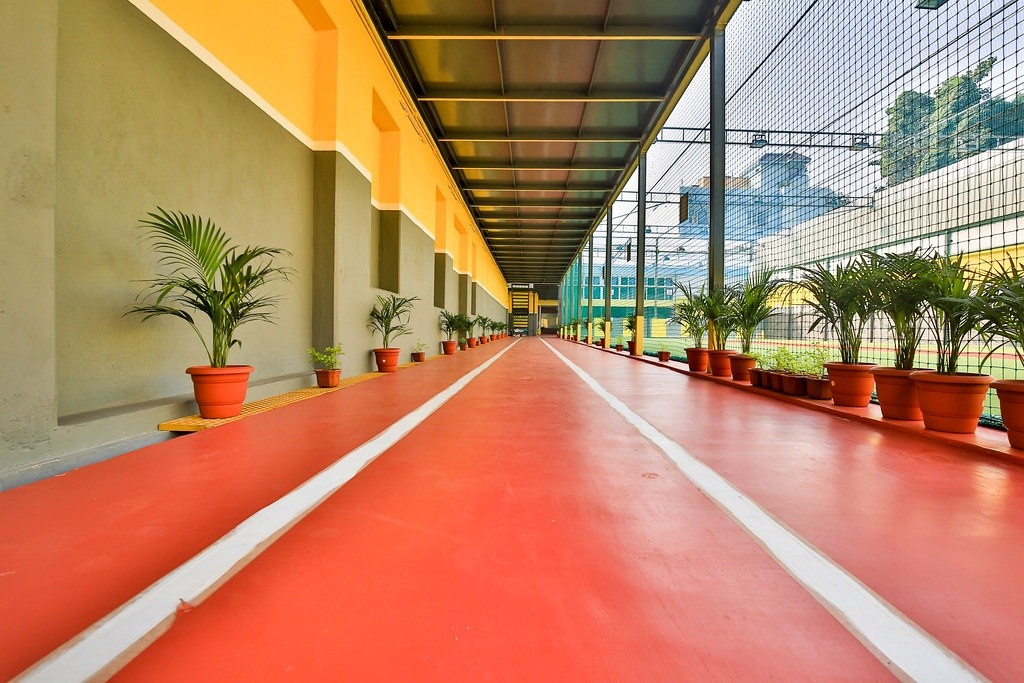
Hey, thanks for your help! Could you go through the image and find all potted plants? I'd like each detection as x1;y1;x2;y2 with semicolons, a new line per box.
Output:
411;344;427;362
367;294;421;372
121;206;299;419
657;243;1024;449
440;310;507;355
616;312;636;355
305;343;345;388
556;313;613;348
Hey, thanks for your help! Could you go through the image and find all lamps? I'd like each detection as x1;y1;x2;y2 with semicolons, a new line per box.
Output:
914;0;950;10
664;257;670;260
749;135;766;148
617;245;622;250
678;246;685;252
850;137;869;151
645;230;651;233
833;197;850;207
